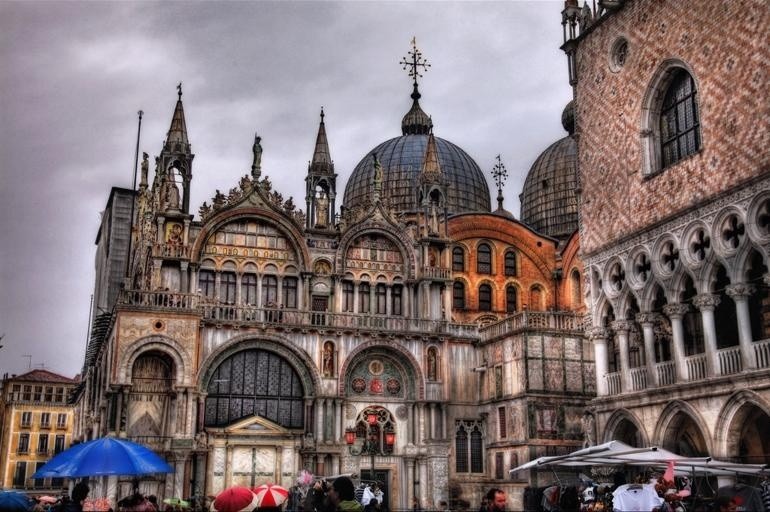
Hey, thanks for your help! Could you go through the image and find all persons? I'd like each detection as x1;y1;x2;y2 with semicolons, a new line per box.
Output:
120;492;201;511
485;488;509;511
361;497;383;511
29;496;60;512
438;499;452;512
305;474;364;511
47;479;89;512
713;495;739;511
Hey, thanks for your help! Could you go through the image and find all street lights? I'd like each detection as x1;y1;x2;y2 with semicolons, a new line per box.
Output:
345;407;398;512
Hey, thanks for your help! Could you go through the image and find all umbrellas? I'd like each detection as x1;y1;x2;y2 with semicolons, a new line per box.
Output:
252;480;290;507
0;488;34;512
209;485;258;511
28;432;179;480
